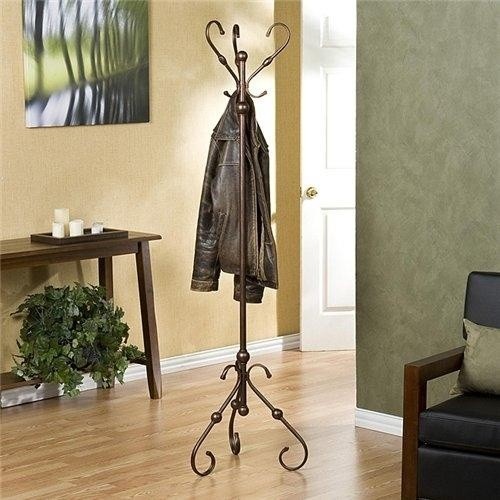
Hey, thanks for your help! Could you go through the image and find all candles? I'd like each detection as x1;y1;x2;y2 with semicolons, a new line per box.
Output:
51;208;104;239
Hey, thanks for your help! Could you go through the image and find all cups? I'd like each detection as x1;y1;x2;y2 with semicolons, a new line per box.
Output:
52;208;84;238
92;222;104;234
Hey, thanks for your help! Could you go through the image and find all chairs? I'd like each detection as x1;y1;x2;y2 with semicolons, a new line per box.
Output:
399;270;500;500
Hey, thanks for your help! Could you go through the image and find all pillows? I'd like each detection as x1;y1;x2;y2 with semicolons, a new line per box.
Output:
446;314;500;398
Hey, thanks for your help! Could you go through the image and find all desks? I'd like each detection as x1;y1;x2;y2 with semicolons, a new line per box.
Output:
0;230;166;401
